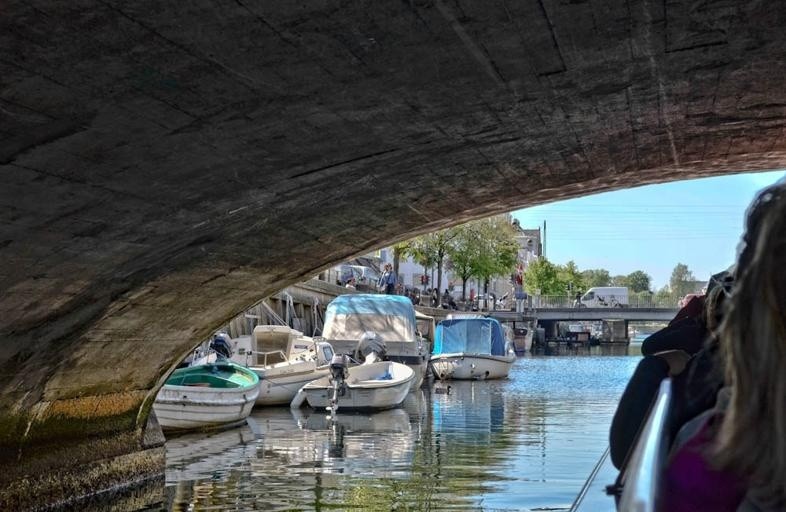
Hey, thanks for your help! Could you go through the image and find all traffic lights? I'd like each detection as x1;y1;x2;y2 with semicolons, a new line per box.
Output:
419;275;431;285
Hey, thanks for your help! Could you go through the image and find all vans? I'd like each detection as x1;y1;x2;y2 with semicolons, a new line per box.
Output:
574;287;629;308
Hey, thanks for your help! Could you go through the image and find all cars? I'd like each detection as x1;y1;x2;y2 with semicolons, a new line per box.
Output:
679;293;703;307
335;264;379;286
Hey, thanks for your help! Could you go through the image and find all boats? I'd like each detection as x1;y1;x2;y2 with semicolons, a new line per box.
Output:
165;417;255;489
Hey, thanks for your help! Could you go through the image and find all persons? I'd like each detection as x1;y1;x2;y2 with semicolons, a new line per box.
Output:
378;262;396;294
344;279;356;291
641;179;786;512
399;283;479;312
